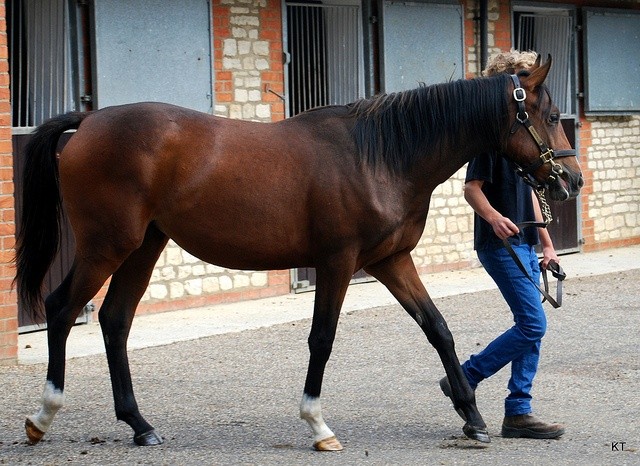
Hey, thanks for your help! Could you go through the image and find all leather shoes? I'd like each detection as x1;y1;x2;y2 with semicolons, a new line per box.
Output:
502;415;565;438
439;376;467;420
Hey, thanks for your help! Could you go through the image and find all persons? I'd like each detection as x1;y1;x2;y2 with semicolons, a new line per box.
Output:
440;46;565;439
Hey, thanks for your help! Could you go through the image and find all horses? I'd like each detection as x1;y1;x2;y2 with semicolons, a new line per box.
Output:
4;51;586;453
